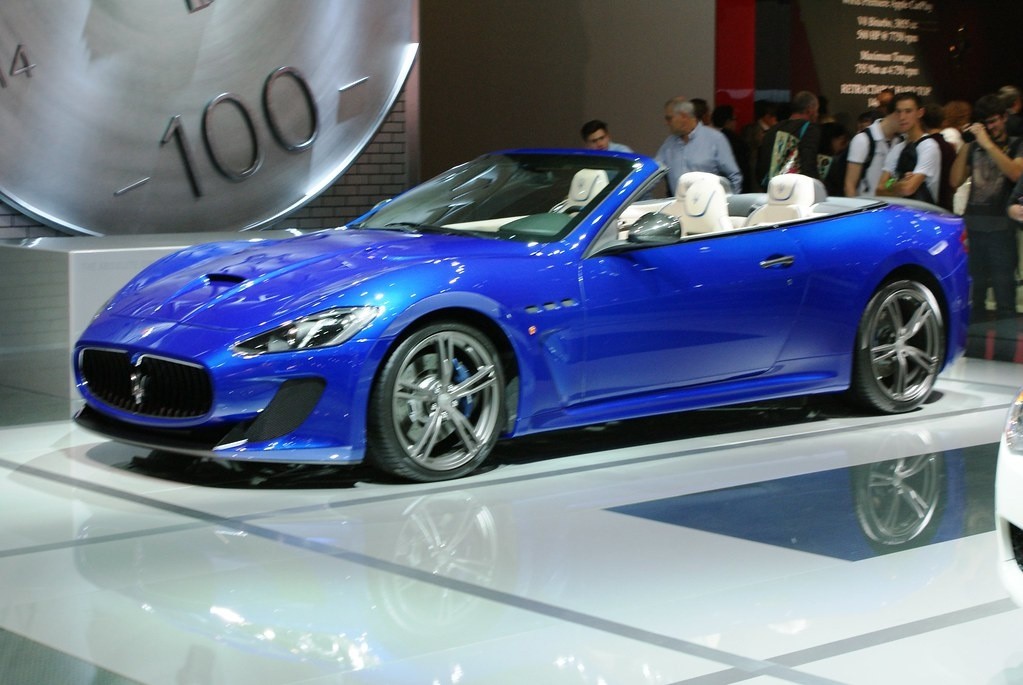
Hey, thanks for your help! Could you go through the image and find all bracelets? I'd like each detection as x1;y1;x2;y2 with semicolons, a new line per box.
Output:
886;178;895;192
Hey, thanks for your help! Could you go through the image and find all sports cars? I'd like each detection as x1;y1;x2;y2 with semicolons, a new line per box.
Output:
70;147;974;491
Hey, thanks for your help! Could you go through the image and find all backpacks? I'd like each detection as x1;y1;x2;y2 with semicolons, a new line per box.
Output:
910;133;956;210
824;127;876;197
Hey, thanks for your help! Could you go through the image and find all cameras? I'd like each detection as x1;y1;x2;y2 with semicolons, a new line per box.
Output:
961;130;976;143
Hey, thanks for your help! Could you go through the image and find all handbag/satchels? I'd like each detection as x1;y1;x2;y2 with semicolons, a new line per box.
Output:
762;122;832;183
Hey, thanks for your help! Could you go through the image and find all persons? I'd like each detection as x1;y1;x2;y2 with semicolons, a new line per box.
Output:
962;324;1018;536
581;120;635;178
688;86;1023;322
655;96;742;197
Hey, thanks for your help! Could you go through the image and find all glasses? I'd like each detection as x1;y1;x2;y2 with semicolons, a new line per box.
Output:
587;134;607;143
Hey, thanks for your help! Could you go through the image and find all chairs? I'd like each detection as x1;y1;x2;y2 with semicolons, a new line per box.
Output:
659;171;829;242
550;169;619;255
